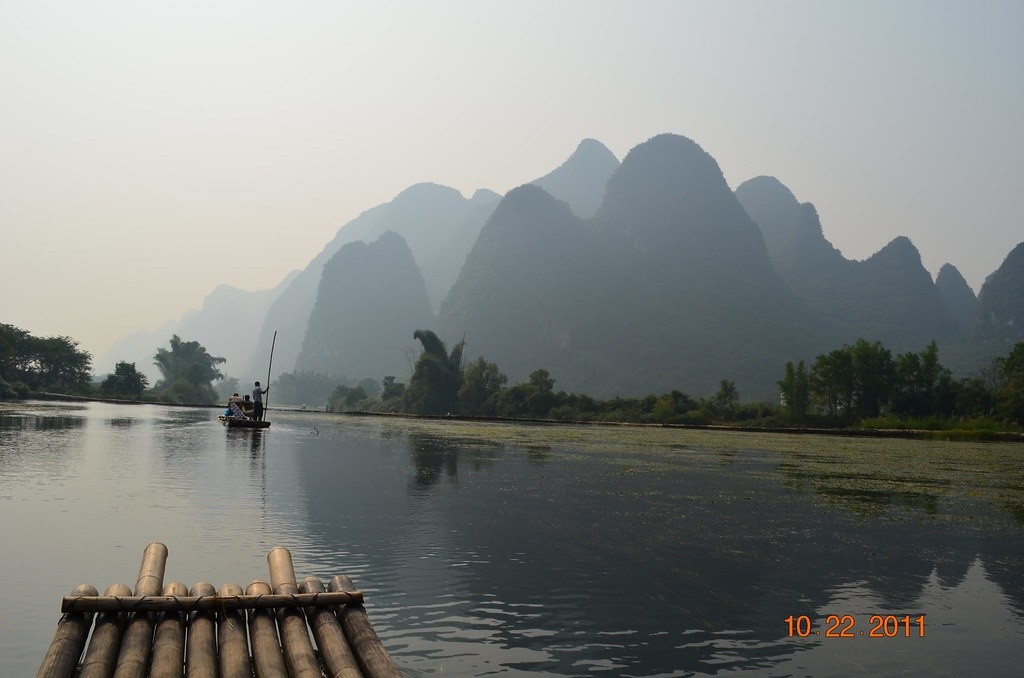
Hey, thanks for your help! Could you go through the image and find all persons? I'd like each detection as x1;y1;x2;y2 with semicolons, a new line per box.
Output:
252;381;269;421
228;393;252;420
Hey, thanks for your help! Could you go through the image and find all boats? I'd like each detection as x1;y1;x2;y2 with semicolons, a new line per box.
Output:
217;416;271;429
